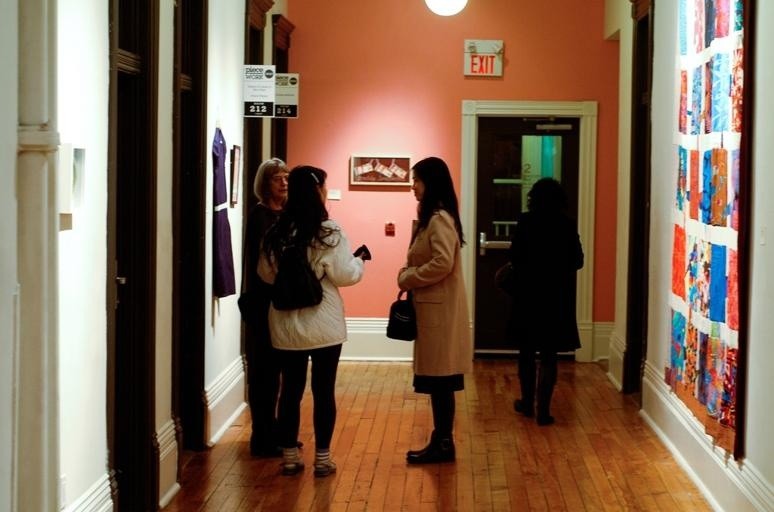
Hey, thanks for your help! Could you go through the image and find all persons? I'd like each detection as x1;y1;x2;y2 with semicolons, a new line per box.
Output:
238;157;304;458
394;156;472;464
503;177;584;426
255;165;365;477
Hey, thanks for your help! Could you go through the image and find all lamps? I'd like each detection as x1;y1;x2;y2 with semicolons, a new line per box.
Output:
425;0;468;17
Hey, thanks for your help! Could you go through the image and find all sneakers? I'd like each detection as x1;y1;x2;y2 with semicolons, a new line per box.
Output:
251;436;302;458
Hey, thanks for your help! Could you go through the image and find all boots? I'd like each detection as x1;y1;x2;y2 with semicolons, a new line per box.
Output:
537;376;554;424
407;430;455;463
515;366;535;416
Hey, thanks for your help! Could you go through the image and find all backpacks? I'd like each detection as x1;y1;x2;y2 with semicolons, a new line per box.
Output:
271;246;322;309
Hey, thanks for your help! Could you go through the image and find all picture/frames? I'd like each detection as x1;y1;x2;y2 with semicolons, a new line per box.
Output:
349;155;413;186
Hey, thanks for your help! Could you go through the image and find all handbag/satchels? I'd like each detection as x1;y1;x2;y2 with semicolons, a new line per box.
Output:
495;262;516;307
387;300;416;341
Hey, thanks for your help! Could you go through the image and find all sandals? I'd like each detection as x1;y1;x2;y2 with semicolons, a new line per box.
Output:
313;462;337;478
281;459;304;475
